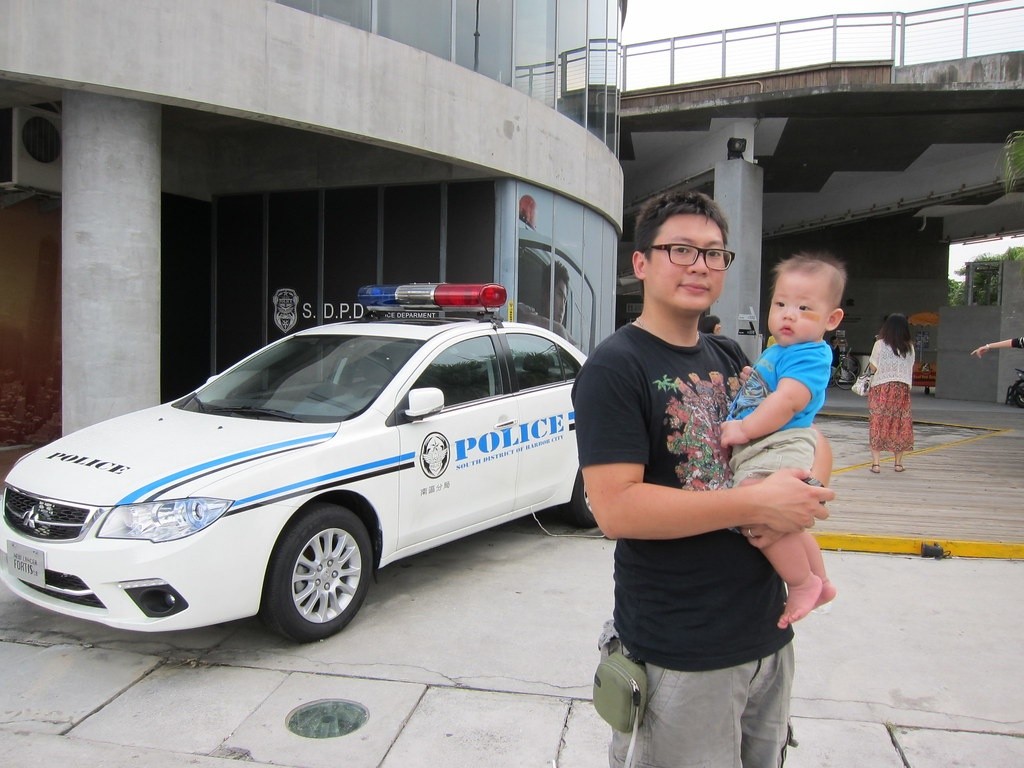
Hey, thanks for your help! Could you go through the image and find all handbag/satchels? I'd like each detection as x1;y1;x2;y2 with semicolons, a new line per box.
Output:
592;652;647;734
851;362;877;396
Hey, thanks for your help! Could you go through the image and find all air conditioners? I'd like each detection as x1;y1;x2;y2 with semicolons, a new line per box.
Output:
0;103;66;199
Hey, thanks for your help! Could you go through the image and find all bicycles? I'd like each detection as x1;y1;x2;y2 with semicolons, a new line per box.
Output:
826;345;860;390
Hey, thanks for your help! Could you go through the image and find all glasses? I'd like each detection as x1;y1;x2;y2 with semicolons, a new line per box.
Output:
651;243;736;270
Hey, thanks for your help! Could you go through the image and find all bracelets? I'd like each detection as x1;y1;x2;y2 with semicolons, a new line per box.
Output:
986;344;990;351
804;476;826;505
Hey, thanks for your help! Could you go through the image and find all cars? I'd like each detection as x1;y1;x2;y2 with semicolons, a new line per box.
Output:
0;284;599;644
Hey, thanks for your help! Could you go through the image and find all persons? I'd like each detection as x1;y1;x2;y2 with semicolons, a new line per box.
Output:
569;189;835;768
700;316;720;334
869;313;916;473
517;261;578;346
719;253;846;630
970;337;1024;359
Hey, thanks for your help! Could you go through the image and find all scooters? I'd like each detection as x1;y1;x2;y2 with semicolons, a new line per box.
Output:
1010;368;1024;408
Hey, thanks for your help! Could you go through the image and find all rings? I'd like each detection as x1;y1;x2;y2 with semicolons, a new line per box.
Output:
748;529;754;538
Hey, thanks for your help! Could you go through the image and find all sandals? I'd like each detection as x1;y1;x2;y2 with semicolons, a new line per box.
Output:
869;464;880;473
895;464;905;472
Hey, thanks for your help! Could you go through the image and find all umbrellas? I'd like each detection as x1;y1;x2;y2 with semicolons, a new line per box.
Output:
908;312;939;362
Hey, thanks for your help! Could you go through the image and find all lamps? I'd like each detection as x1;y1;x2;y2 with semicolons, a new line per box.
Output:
726;137;747;160
